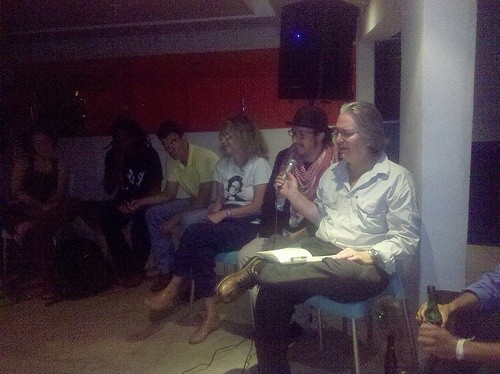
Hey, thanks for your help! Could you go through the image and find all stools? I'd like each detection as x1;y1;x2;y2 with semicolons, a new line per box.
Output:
3;229;63;277
307;262;419;374
214;251;256;329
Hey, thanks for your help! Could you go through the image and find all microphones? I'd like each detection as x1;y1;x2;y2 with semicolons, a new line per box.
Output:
276;159;298;192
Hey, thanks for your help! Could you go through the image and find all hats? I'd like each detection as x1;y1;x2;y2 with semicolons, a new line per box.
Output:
285;106;332;134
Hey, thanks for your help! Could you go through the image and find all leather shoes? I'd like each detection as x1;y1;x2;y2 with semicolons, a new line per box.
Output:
216;256;265;303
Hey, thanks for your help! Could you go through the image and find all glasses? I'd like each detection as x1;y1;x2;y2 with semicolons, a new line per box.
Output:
288;129;317;139
332;127;362;138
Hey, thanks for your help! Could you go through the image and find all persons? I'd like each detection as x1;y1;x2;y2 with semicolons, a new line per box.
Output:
145;112;273;344
216;100;422;374
415;262;500;374
0;122;74;299
238;105;340;347
97;115;163;287
117;120;220;290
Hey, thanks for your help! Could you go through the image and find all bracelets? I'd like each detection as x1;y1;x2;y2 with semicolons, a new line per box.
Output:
456;338;465;362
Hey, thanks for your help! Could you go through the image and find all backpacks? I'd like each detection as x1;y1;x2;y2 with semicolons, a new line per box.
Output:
56;237;109;299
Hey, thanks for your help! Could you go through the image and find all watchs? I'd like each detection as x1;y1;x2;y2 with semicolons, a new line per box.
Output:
227;208;232;218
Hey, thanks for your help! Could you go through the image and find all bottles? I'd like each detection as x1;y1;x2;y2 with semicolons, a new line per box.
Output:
424;285;442;328
384;336;397;374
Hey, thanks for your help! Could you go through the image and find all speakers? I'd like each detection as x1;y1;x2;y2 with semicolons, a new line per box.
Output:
276;0;360;101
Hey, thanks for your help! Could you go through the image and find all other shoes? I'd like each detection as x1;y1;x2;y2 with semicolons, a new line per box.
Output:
150;275;170;293
188;315;218;344
126;262;144;286
148;285;179;310
